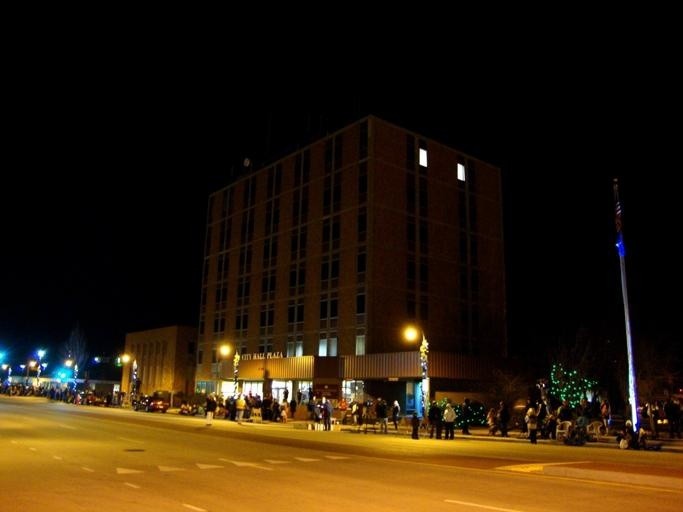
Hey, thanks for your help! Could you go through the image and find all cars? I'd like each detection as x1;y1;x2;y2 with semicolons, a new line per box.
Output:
132;396;170;414
5;381;110;407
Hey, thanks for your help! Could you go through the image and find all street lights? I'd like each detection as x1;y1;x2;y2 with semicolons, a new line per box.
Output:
0;347;50;385
122;351;139;401
403;323;431;435
221;343;239;401
64;359;79;390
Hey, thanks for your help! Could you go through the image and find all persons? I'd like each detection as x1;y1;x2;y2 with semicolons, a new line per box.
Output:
0;381;111;407
351;398;400;434
411;399;508;440
524;394;683;451
178;387;333;431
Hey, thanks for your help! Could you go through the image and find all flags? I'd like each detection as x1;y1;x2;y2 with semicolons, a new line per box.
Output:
614;189;622;234
